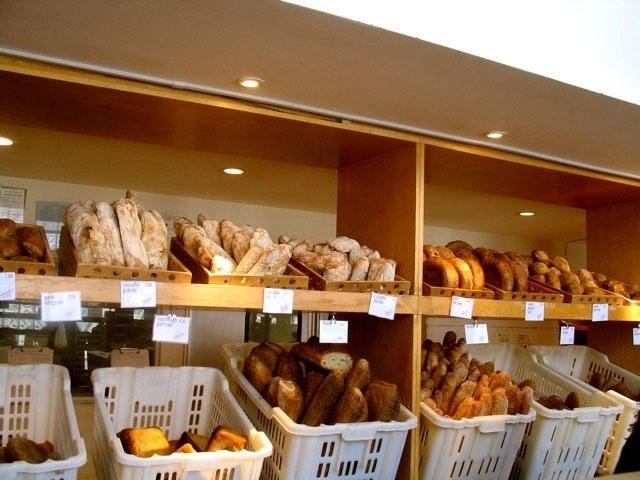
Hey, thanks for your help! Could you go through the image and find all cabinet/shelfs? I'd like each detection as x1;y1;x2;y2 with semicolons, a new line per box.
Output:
0;42;640;479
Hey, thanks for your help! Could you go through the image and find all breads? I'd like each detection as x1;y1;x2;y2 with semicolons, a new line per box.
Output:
432;362;446;389
560;272;580;287
233;246;262;275
92;201;126;266
277;233;289;243
466;400;485;418
345;358;371;392
520;255;550;274
207;426;247;479
365;380;400;421
446;344;461;364
5;438;47;464
288;239;300;248
291;342;353;372
445;241;475;254
470;358;480;369
597;272;609;288
179;224;207;256
314;245;331;254
577;269;597;288
551;256;570;272
174;217;195;237
293;244;309;260
0;238;21;259
252;340;287;375
242;353;272;395
368;259;396;281
64;201;109;266
350;246;380;266
448;258;473;289
323;251;352;282
423;257;459;287
0;219;17;238
176;431;208;451
305;372;326;408
504;251;529;292
536;394;565;410
518;379;533;391
461;353;473;368
472;375;491;399
246;243;293;276
456;338;466;355
328;236;360;252
452;397;474;420
349;257;368;281
434;389;443;407
331;387;368;423
506;385;521;415
451;362;469;381
140;210;168;270
478;393;492;415
193;237;238;274
426;351;438;371
423;245;440;257
608;279;636;299
305;254;331;273
548;267;562;290
467;365;480;383
520;385;533;415
249;228;274;248
21;236;45;258
565;392;580;410
197;213;221;246
420;371;434;400
488;372;512;392
300;240;313;251
456;248;485;289
588;372;603;391
532;250;548;263
491;388;508;415
429;342;445;362
112;198;149;269
530;275;546;284
448;379;476;417
609;383;639;401
300;370;346;427
441;373;459;415
567;281;584;295
424;398;444;416
443;330;457;346
435;246;456;258
116;426;171;457
176;443;197;452
220;218;238;255
264;376;304;423
273;352;306;384
479;361;495;375
473;248;515;290
16;226;41;239
233;226;255;264
586;287;604;295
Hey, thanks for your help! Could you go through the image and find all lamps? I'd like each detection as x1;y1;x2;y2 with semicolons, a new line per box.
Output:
236;76;266;92
218;166;244;177
0;133;14;149
482;129;508;143
517;209;536;219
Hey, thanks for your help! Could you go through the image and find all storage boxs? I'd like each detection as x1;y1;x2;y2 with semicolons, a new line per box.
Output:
420;391;537;480
219;339;418;480
0;361;88;480
525;343;640;476
461;339;624;477
89;364;272;479
